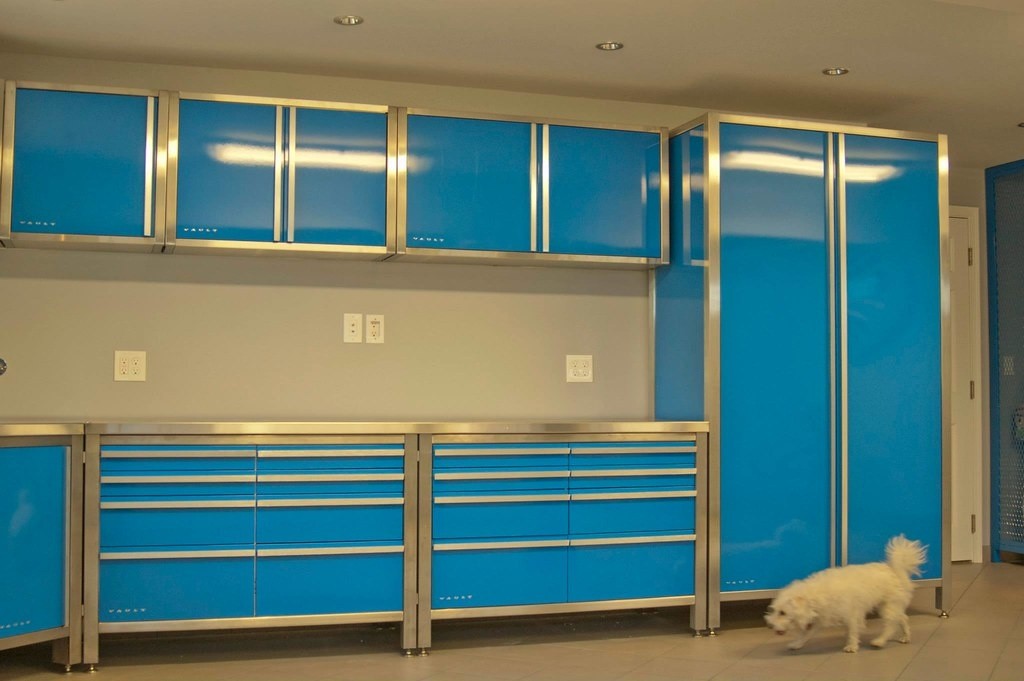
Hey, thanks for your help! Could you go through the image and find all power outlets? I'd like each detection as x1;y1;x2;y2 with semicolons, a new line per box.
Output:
566;354;593;383
365;314;385;344
114;350;147;381
343;313;362;343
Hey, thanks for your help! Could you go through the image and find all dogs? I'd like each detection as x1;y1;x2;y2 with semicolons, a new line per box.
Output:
762;534;929;652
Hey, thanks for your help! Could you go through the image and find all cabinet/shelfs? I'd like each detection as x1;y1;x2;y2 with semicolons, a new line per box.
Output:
650;110;949;620
0;421;84;673
383;106;670;272
84;421;417;675
161;90;396;263
414;418;708;657
0;79;164;255
